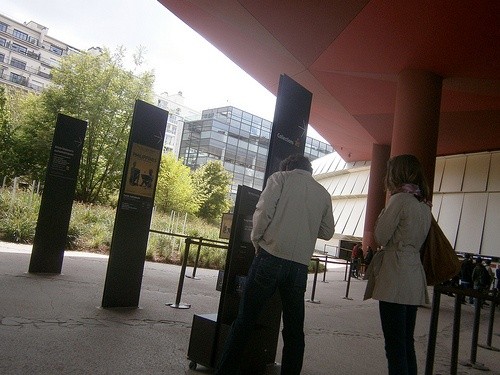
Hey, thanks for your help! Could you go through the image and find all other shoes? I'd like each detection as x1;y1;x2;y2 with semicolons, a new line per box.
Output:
351;274;357;278
461;300;468;305
484;302;490;305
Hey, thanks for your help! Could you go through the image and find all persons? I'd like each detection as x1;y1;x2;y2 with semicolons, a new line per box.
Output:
216;155;335;375
362;155;432;375
351;243;381;280
442;253;500;309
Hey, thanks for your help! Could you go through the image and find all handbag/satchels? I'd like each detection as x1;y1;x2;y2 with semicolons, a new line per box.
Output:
419;212;460;286
